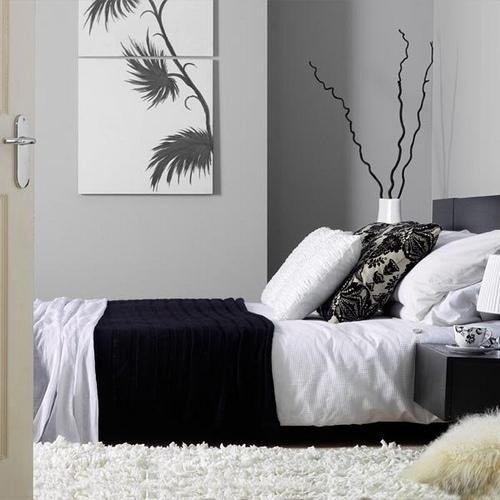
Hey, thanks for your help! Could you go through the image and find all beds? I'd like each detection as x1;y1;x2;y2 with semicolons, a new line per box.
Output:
31;188;498;446
31;408;499;500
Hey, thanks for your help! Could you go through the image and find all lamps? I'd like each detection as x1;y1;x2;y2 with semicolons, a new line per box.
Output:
475;254;499;321
376;198;403;226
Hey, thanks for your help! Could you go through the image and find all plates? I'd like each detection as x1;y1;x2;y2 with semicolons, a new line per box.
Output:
446;344;495;354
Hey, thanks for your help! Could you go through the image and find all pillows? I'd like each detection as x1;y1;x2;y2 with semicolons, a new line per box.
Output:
259;218;499;325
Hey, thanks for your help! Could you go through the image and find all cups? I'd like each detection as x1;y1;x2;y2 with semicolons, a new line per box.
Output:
453;324;493;345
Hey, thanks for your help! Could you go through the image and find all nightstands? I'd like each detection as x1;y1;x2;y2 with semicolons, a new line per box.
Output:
410;342;499;446
